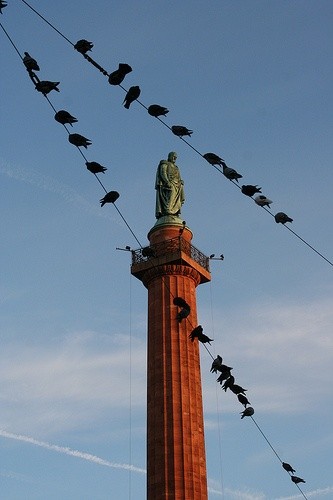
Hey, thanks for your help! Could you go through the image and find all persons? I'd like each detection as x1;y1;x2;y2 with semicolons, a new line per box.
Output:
154;152;185;218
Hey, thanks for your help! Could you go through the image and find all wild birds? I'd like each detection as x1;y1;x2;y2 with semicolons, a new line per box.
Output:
100;190;120;207
86;161;108;174
69;133;92;149
240;407;254;419
74;39;94;53
241;185;263;197
275;212;293;224
203;152;224;167
282;462;296;474
252;192;272;209
108;63;132;85
221;162;243;183
54;110;78;127
172;296;191;322
23;51;40;72
171;125;193;139
148;104;170;118
188;325;214;345
35;80;60;95
291;475;306;484
121;85;141;110
209;356;250;408
